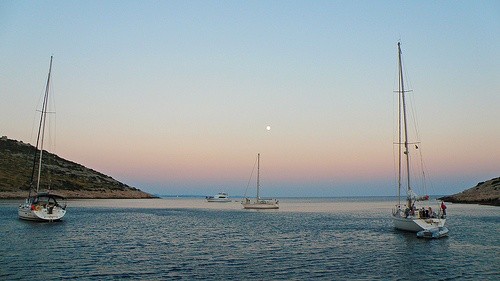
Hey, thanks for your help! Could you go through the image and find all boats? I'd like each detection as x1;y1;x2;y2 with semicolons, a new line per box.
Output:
205;191;238;202
416;227;450;239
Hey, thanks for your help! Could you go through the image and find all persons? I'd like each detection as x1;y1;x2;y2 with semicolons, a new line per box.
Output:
35;204;42;211
396;200;447;218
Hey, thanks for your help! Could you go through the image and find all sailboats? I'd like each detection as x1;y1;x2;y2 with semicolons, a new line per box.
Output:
392;41;446;232
241;153;279;209
18;56;70;222
